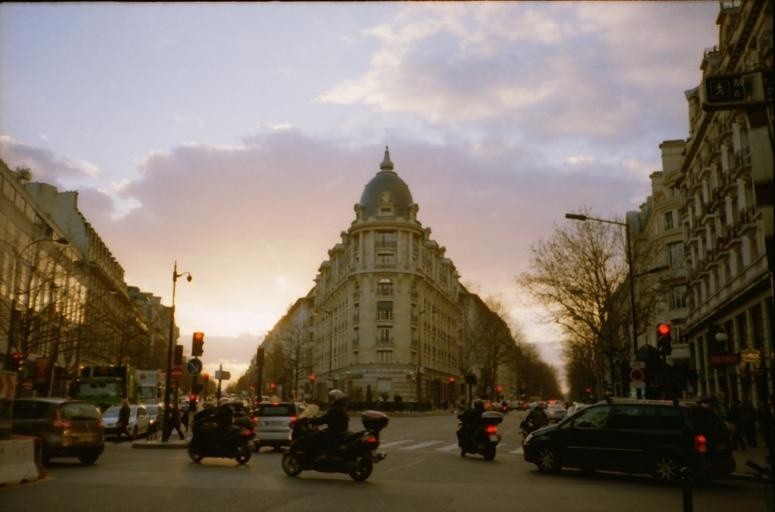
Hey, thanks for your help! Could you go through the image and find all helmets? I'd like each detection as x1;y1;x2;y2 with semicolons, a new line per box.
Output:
218;397;231;408
473;399;484;408
328;389;345;405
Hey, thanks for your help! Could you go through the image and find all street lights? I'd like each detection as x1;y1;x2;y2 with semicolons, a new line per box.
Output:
165;261;191;415
565;215;641;398
4;239;68;369
715;330;729;406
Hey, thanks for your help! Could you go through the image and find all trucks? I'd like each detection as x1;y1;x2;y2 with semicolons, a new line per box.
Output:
80;365;166;411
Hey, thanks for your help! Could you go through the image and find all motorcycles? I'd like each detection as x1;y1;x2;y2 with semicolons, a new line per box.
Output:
282;410;387;481
188;408;251;464
456;399;503;460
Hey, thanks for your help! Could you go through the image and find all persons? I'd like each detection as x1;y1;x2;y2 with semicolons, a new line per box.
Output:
191;395;241;449
461;398;485;449
561;400;575;417
522;400;546;431
304;388;350;466
698;391;775;453
117;394;190;443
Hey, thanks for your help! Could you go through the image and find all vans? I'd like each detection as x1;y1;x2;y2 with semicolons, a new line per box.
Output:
0;397;103;468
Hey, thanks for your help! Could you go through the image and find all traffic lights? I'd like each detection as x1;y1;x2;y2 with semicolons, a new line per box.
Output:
656;323;671;355
192;332;204;356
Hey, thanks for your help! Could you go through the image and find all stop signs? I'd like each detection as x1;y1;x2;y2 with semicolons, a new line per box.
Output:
171;367;184;379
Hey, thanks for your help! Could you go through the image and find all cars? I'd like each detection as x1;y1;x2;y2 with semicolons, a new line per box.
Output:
102;402;164;441
511;398;736;483
253;402;296;451
